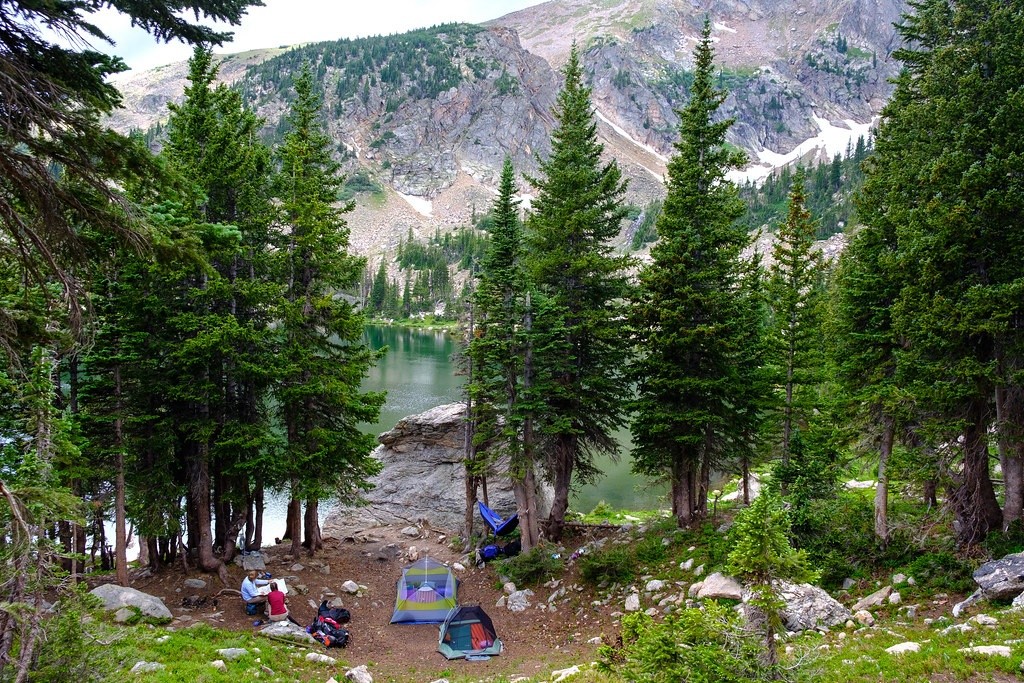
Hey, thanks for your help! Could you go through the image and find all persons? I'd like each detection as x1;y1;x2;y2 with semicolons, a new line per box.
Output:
267;581;289;623
240;570;273;616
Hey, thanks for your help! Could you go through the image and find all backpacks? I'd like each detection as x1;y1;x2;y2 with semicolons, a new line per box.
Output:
479;544;503;562
307;600;350;648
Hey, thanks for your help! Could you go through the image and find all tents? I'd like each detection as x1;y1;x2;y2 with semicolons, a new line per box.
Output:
389;556;463;625
436;604;504;660
